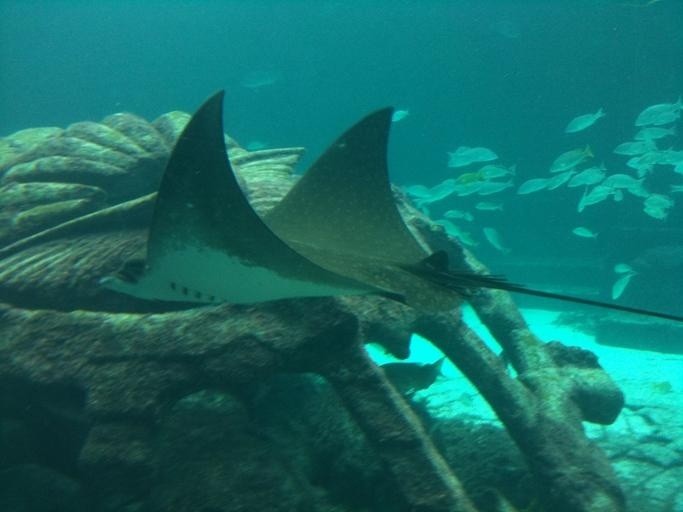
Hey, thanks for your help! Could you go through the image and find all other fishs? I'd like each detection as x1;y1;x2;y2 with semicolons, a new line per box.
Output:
573;227;599;241
391;144;515;253
516;93;683;221
